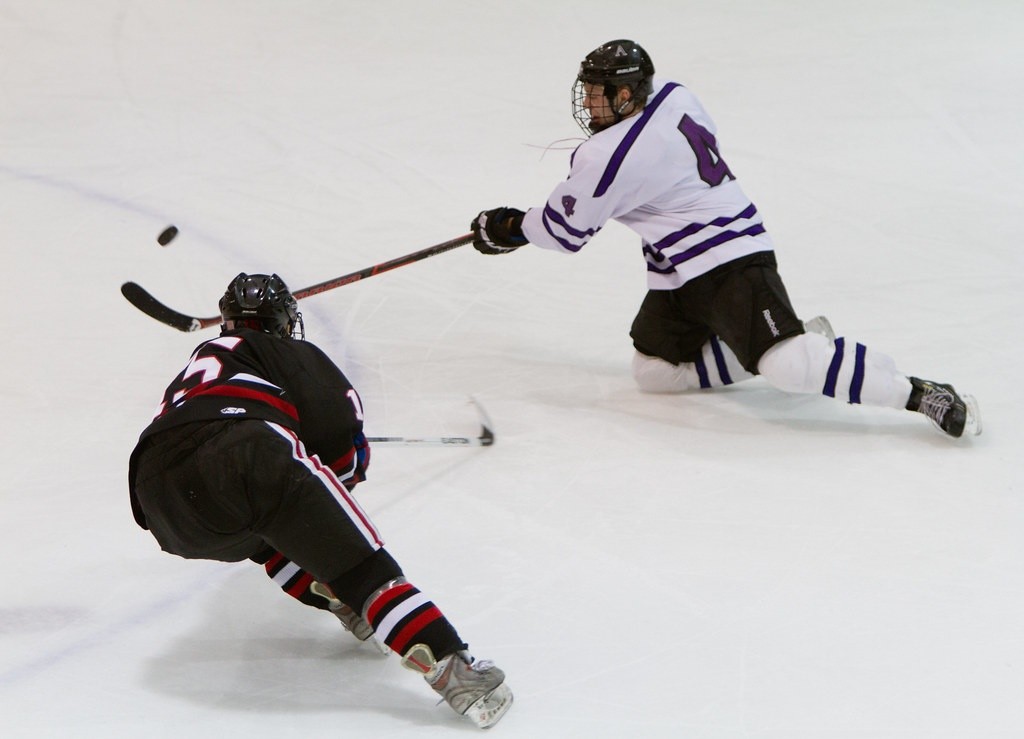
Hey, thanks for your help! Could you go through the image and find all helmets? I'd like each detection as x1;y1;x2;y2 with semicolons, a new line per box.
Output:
220;271;299;336
572;39;658;138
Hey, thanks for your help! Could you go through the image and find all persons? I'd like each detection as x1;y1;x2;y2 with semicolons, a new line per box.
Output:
471;39;981;441
129;273;514;730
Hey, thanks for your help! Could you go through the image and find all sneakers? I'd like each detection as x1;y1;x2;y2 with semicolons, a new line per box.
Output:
425;649;515;730
325;594;393;660
801;314;836;347
909;377;985;440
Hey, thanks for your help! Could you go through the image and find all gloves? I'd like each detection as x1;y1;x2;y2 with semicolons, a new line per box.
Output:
470;205;529;257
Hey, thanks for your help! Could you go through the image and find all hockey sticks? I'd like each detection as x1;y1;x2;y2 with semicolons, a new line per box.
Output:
117;231;478;334
365;391;497;448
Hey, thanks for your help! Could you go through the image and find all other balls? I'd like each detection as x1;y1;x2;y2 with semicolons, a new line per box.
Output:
158;224;179;245
